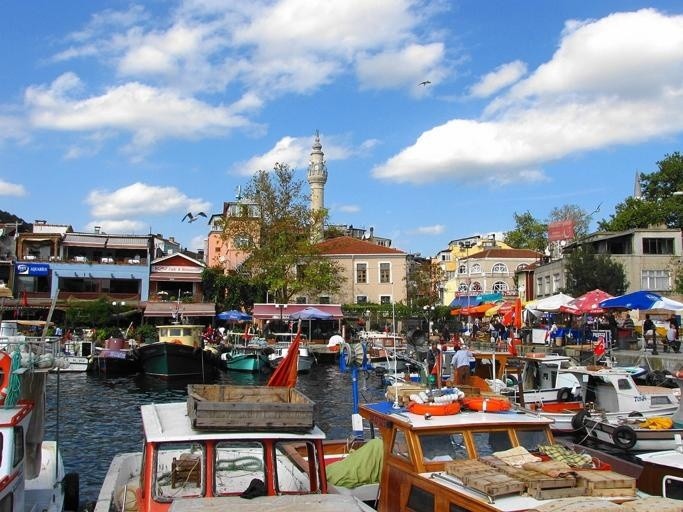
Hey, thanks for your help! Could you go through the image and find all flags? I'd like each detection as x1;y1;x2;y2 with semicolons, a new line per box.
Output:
267;324;301;386
22;289;27;305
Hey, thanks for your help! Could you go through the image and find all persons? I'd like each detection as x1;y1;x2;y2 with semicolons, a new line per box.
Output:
54;326;97;341
321;322;328;343
427;342;440;373
451;345;474;384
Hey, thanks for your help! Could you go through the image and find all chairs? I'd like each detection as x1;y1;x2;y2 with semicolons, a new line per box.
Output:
168;455;202;490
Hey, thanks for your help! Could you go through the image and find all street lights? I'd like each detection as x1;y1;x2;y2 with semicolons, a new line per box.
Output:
457;242;471;307
216;255;241;273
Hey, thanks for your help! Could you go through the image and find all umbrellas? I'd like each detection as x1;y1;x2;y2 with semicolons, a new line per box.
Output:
289;307;333;342
218;309;252;328
450;289;683;351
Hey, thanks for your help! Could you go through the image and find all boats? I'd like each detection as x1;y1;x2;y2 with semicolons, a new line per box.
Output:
265;320;315;376
281;368;683;512
0;317;82;512
220;322;269;374
0;319;95;373
89;384;379;512
627;433;683;503
138;288;221;384
85;323;139;379
287;287;682;450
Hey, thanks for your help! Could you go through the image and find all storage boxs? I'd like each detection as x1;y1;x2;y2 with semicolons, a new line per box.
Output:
185;382;317;434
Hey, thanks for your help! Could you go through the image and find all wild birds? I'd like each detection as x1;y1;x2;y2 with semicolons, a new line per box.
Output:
417;80;430;87
590;200;604;216
181;212;207;224
